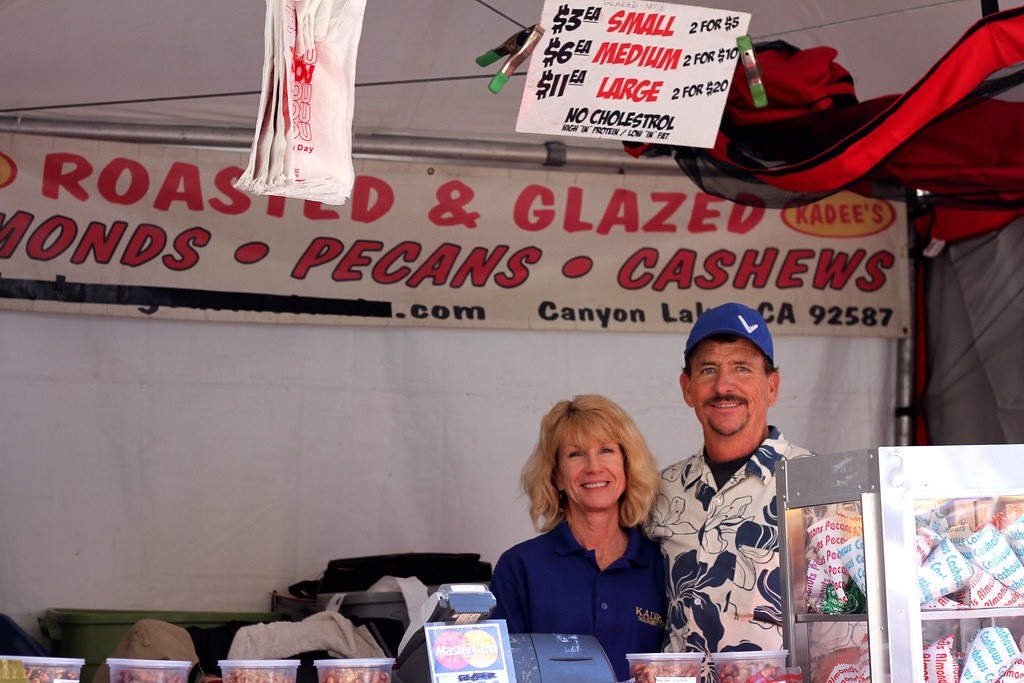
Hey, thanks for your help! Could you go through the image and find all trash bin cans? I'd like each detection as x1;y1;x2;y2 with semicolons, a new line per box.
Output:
38;608;291;683
0;612;49;656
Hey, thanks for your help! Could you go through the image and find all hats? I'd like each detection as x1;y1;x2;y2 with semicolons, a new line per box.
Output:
683;303;773;365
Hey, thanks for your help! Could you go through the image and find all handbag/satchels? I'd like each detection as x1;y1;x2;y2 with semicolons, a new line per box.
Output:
288;551;493;598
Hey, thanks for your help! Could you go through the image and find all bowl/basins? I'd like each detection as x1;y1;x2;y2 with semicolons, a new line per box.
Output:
313;658;396;683
0;655;86;683
625;652;706;683
217;659;301;683
711;649;789;683
105;657;192;683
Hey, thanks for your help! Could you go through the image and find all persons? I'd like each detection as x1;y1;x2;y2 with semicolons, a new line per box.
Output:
640;305;822;683
486;394;669;683
